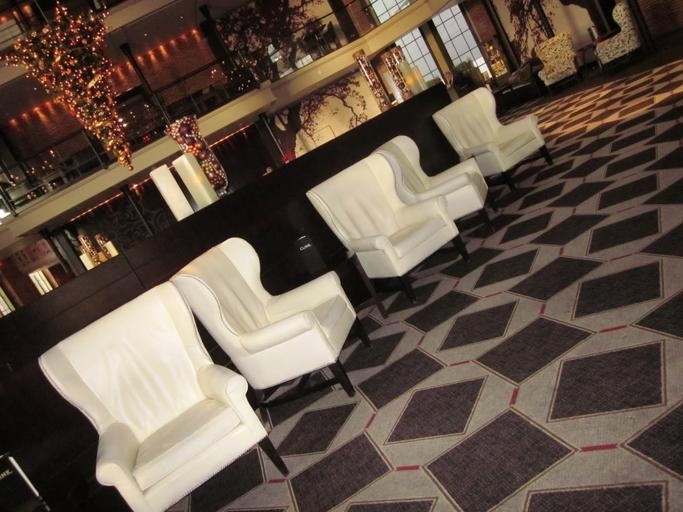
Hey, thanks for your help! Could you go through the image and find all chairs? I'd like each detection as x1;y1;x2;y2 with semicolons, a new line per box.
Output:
36;279;288;512
305;153;469;305
312;22;340;54
429;85;553;195
591;1;648;74
169;236;370;401
369;134;498;234
532;31;585;99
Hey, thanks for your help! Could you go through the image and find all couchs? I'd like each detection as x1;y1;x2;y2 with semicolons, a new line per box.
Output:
496;58;544;105
